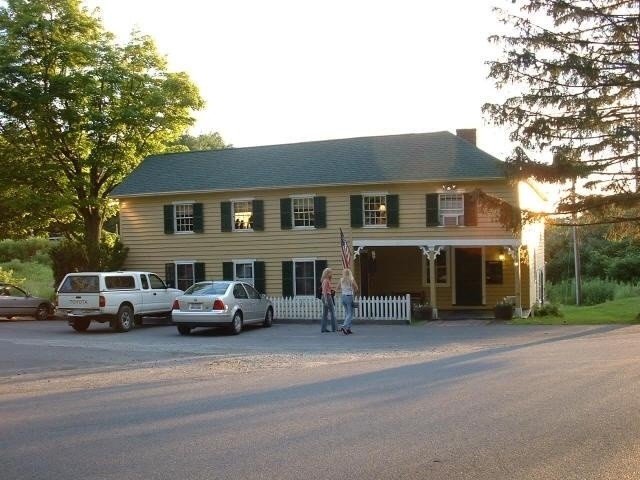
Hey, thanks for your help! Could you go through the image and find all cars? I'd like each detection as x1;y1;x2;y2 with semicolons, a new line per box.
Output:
0;283;55;321
56;271;273;335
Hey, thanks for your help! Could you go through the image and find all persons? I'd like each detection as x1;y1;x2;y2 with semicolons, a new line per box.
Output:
336;268;360;335
320;268;340;333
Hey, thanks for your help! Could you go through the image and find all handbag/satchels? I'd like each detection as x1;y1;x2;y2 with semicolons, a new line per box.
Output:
352;300;359;308
317;287;322;299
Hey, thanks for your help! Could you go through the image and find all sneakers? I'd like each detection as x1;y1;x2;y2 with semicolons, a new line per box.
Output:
321;327;351;335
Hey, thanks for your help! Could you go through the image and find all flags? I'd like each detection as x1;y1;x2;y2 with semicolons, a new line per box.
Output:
340;231;350;270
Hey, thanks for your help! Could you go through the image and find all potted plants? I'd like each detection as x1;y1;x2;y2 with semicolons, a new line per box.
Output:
414;301;432;320
493;299;514;320
